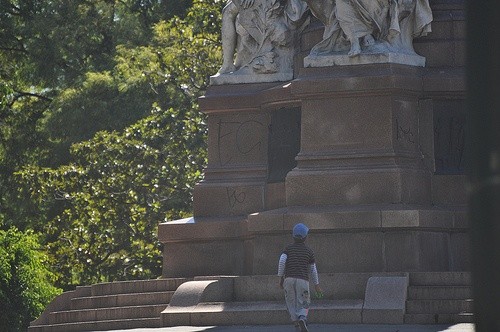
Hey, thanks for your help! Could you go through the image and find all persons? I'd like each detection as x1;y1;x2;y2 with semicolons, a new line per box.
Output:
277;223;324;332
209;0;433;86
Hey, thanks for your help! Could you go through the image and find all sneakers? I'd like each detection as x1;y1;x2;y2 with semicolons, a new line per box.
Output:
295;321;301;332
298;316;309;332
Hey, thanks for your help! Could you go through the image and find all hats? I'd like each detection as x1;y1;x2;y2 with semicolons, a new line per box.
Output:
293;223;309;240
